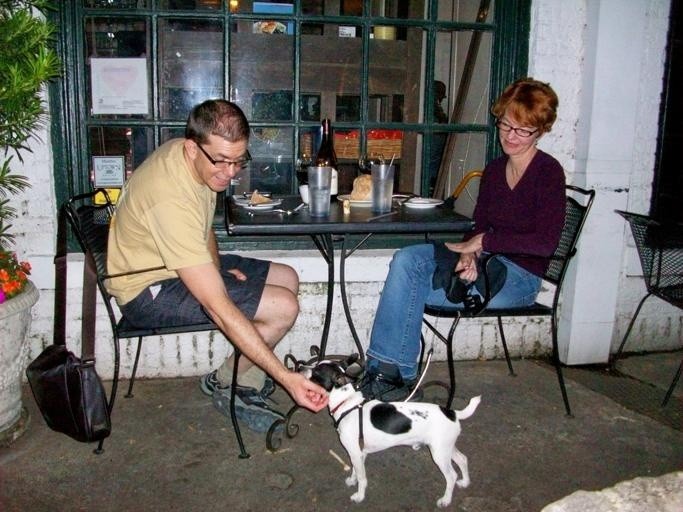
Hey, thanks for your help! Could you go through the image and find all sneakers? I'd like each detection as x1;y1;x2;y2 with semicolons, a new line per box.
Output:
350;370;411;398
202;370;274;399
210;387;286;434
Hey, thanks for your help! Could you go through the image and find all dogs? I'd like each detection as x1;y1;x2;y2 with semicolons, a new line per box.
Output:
296;353;483;507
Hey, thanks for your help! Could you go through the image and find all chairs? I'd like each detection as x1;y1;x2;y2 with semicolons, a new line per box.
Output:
416;184;595;419
614;210;683;407
65;188;251;458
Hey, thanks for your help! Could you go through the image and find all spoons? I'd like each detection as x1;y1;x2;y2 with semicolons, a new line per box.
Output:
250;203;303;216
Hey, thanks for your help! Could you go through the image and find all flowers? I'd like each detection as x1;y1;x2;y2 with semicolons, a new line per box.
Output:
1;249;32;304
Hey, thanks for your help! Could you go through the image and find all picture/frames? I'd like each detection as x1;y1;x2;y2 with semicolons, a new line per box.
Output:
92;155;125;188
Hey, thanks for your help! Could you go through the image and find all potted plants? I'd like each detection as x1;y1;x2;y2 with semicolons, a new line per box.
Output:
1;0;71;434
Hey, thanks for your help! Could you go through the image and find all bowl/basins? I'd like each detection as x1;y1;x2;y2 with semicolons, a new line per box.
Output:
300;185;311;205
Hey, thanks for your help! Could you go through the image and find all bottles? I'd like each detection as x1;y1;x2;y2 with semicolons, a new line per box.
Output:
316;119;337;202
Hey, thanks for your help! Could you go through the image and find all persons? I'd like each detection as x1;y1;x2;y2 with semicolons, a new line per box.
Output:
355;79;566;402
102;98;330;432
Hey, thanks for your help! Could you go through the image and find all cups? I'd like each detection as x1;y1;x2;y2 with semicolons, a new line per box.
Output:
308;166;330;217
371;165;394;212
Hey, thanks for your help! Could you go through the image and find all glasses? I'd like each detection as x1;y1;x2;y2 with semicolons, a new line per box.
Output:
189;142;252;168
497;120;543;138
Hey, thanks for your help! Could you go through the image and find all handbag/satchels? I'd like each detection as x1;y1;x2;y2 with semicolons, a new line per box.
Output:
25;200;111;445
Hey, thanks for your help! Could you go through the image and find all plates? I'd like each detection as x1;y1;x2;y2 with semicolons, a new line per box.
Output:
392;194;411;203
402;196;443;209
338;194;374;208
235;200;280;209
